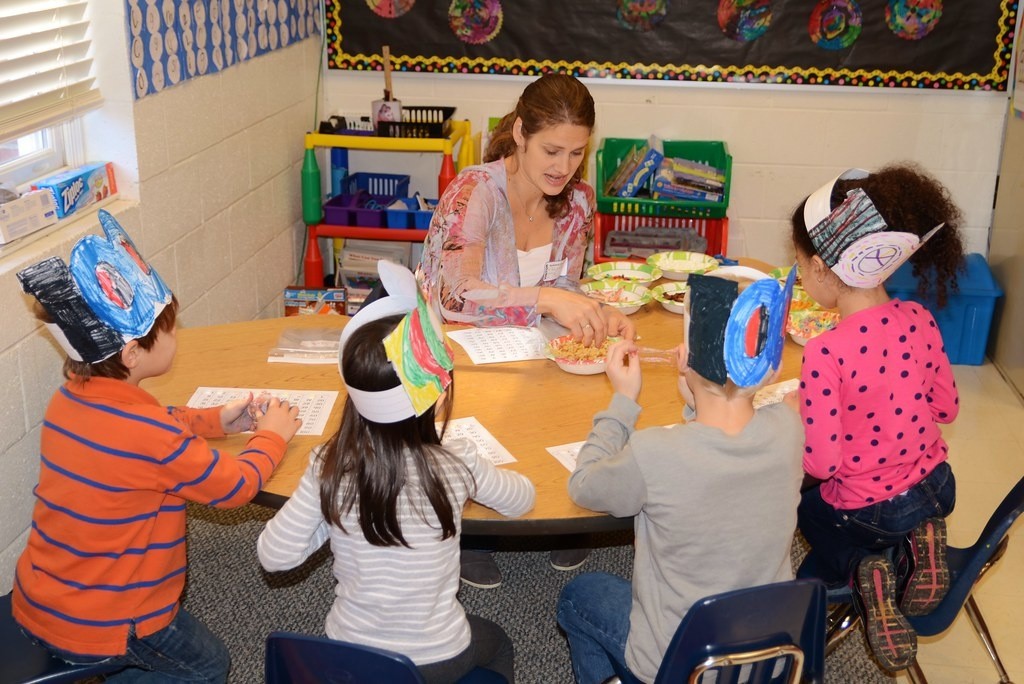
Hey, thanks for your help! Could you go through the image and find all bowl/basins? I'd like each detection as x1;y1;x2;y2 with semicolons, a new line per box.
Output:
652;282;686;314
544;334;625;374
580;281;652;315
767;266;841;346
645;251;719;281
586;261;662;287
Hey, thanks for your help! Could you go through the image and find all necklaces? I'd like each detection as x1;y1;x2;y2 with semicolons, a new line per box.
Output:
517;191;540;221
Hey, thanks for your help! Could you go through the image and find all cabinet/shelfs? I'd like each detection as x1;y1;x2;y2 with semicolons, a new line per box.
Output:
307;116;475;288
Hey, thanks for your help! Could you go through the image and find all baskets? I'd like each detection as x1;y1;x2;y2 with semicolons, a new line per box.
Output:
595;138;733;263
321;106;456;231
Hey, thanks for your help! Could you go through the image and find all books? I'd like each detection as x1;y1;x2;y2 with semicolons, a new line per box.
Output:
267;327;343;364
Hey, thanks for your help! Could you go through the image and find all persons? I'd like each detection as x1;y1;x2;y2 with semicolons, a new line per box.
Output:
556;275;805;684
376;104;395;121
790;162;966;671
414;74;637;589
257;314;535;684
11;293;302;684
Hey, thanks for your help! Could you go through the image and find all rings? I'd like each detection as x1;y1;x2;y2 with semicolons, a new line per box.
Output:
581;324;591;329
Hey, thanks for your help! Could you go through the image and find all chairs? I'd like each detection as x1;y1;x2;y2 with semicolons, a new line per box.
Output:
823;474;1024;667
263;631;513;684
615;578;829;684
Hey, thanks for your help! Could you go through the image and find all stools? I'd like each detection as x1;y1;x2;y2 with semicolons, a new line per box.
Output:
0;594;126;684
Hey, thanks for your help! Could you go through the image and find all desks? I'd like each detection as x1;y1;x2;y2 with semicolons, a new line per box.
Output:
136;255;842;554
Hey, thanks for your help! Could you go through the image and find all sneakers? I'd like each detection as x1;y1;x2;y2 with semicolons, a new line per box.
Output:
850;555;917;672
895;516;950;616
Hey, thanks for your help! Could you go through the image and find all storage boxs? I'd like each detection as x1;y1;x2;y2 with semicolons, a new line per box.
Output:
596;137;734;220
24;159;120;219
1;187;60;246
283;265;380;319
595;210;729;263
881;252;1003;364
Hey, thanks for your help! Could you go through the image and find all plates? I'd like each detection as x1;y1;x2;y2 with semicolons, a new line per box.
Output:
704;266;784;292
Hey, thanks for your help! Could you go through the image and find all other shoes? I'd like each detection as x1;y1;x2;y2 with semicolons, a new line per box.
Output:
459;549;502;589
550;549;591;571
